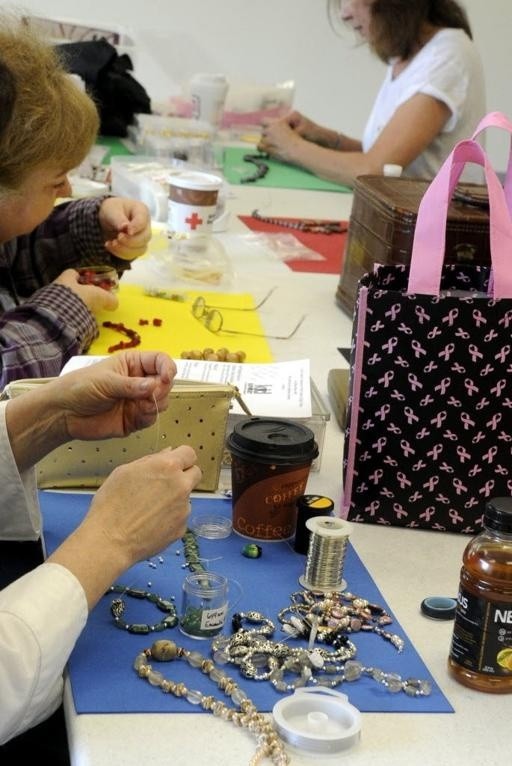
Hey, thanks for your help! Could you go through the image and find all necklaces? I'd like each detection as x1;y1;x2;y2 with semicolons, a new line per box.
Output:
125;635;284;766
102;581;176;636
209;592;434;700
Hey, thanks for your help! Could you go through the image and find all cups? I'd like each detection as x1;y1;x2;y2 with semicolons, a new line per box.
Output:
191;70;230;137
177;573;229;641
225;418;320;542
166;169;222;257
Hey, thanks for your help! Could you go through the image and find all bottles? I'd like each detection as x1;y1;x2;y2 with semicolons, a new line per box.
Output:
446;498;512;693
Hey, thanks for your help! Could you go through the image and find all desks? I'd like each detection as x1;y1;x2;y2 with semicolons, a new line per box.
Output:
0;54;512;766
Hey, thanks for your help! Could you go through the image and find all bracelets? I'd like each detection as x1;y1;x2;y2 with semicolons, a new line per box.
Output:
333;130;343;151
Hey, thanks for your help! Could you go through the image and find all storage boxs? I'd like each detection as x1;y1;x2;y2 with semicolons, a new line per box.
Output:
337;166;493;321
107;152;230;220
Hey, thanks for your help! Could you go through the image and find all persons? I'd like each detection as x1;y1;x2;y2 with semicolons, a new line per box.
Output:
1;350;205;744
256;0;490;191
1;24;154;384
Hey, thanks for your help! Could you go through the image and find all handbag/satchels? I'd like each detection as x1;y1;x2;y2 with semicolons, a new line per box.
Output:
3;375;237;491
51;39;151;137
340;110;512;535
